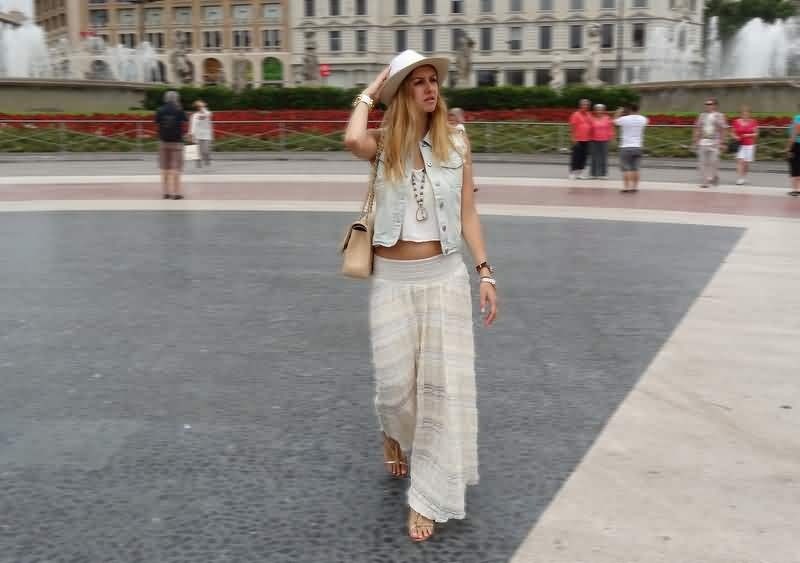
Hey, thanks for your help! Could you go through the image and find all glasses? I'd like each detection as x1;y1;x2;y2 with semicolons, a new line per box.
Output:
704;103;715;106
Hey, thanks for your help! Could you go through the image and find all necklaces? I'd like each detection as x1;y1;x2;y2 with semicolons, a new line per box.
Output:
411;167;428;221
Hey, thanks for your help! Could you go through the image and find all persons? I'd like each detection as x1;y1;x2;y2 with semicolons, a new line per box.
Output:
787;104;800;197
153;91;191;200
729;104;760;185
687;97;728;188
334;50;497;542
188;100;214;168
449;107;478;193
612;99;650;193
567;98;617;181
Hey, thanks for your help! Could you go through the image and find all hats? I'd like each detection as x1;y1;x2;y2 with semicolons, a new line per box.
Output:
379;49;449;104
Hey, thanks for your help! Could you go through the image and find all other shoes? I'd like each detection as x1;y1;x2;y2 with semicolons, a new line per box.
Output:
735;178;745;185
792;191;800;196
164;194;168;198
175;194;183;199
622;188;639;193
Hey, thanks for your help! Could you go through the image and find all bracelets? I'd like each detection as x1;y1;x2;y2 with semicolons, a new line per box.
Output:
351;93;375;113
475;261;497;275
480;277;497;288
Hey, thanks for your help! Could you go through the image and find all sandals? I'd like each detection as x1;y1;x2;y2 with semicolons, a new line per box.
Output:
408;507;435;542
384;437;409;477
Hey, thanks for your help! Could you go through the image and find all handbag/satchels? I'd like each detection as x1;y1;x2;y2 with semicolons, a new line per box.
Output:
341;211;374;278
184;143;201;160
